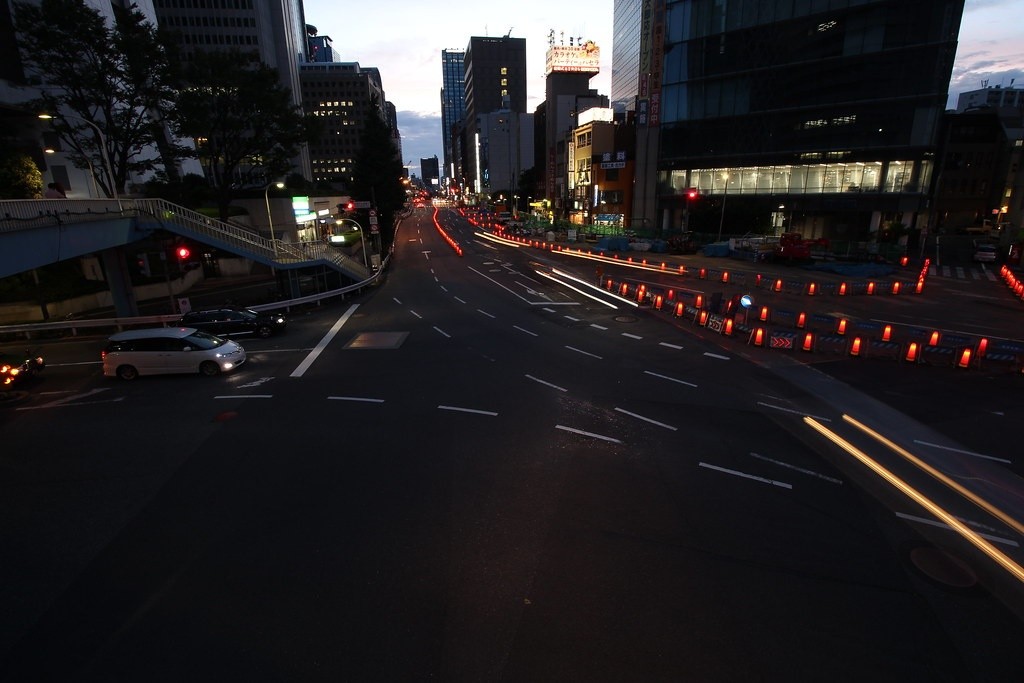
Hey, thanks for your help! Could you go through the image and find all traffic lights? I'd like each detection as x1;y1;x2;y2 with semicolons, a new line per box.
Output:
138;253;150;277
177;247;193;273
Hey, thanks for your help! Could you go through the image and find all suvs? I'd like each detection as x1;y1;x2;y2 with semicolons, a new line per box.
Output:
178;306;288;339
101;327;248;377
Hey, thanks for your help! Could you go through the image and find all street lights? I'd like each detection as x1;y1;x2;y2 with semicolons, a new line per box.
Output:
37;113;119;199
42;147;101;199
717;173;728;243
265;181;285;258
337;219;369;269
